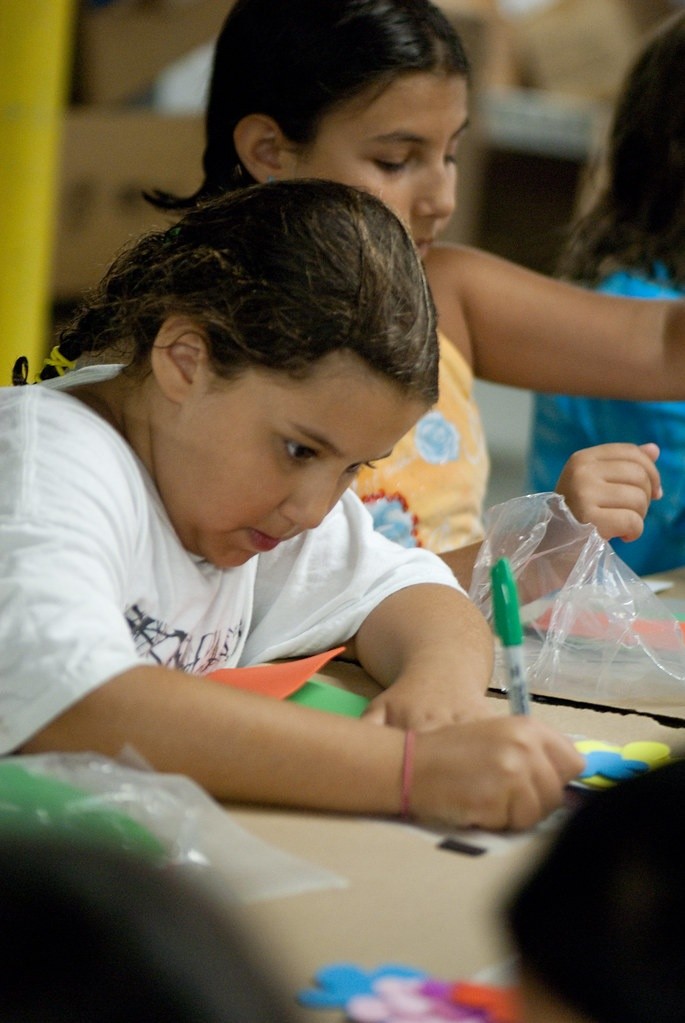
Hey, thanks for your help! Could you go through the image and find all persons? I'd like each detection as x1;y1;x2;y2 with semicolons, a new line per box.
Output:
493;747;685;1022
139;1;684;632
485;16;685;629
0;814;305;1022
1;175;583;827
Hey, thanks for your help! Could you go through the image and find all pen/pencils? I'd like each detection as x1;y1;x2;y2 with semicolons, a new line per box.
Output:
488;559;531;717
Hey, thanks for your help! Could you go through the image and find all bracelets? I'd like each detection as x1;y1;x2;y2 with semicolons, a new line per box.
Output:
402;731;413;815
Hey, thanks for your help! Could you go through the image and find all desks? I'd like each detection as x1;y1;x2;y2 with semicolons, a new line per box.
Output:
83;564;685;1023
